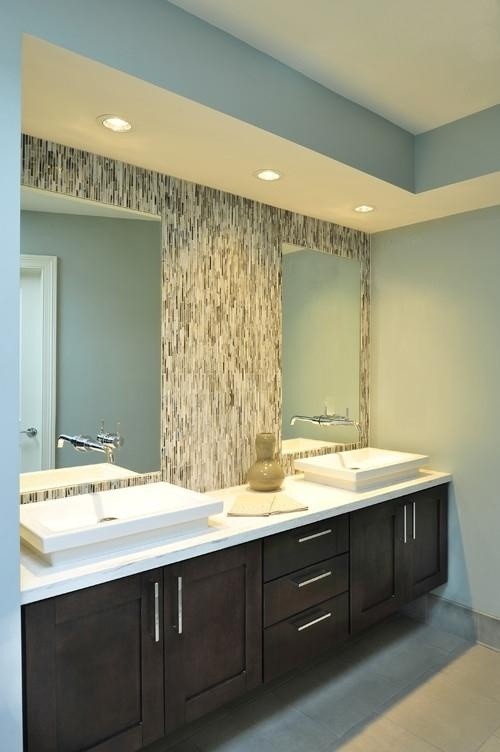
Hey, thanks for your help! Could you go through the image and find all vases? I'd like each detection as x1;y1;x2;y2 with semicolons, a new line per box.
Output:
247;432;285;491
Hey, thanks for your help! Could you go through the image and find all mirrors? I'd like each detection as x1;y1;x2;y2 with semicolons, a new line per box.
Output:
20;183;160;493
282;241;361;455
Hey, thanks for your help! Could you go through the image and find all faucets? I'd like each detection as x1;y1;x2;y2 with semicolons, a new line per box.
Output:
53;419;107;452
75;435;118;465
320;404;366;438
289;406;330;435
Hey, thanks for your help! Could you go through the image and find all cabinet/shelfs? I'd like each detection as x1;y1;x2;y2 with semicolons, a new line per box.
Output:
350;484;447;638
263;515;350;685
22;540;263;752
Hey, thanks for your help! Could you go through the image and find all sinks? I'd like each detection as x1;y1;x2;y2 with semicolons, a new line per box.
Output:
292;445;431;491
20;479;224;566
19;459;140;490
281;436;344;456
